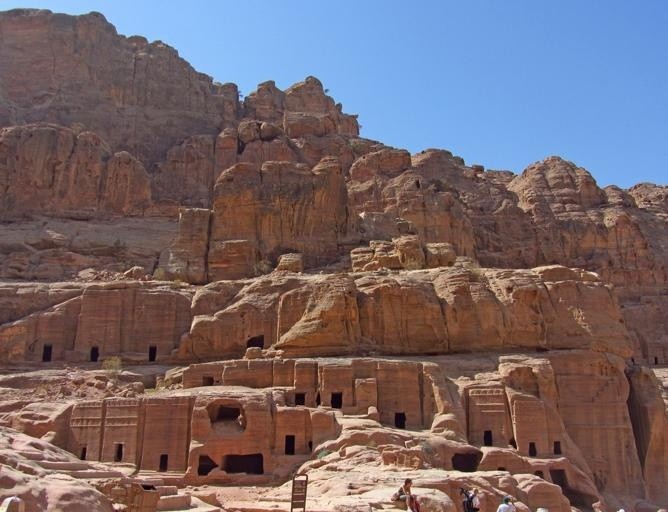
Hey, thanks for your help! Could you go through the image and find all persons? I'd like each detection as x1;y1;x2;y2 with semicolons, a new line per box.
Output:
459;488;470;512
391;477;417;511
495;495;519;512
468;486;482;511
